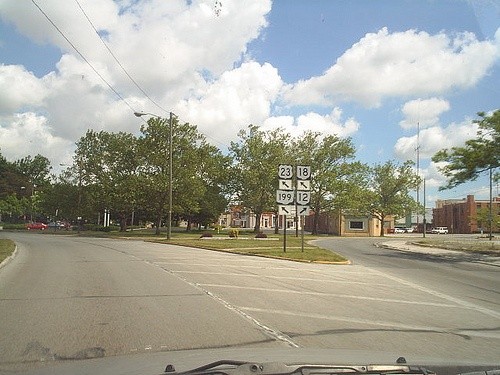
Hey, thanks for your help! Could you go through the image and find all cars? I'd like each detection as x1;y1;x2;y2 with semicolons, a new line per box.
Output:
26;219;71;231
394;222;448;235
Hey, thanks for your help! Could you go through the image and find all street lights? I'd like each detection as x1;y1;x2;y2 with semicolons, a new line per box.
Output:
134;110;174;241
